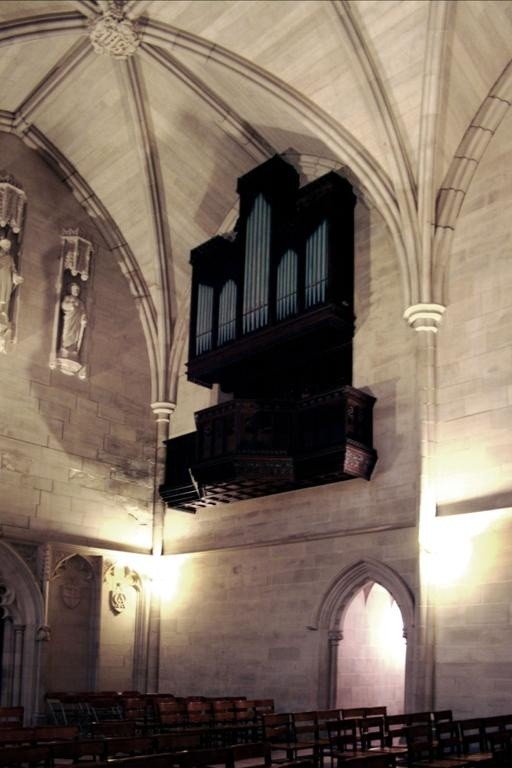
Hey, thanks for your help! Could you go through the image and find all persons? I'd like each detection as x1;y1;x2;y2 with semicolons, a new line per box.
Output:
59;282;90;362
1;237;26;335
